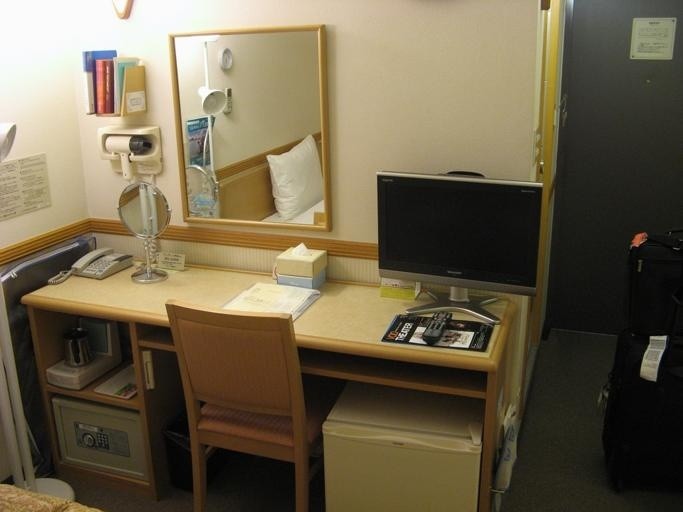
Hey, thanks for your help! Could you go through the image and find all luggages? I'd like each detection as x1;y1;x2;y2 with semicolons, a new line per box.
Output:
602;326;682;491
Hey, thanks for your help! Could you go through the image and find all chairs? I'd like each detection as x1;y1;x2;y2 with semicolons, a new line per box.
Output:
165;296;331;512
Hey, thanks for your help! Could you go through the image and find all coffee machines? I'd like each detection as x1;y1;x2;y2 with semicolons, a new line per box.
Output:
45;316;122;391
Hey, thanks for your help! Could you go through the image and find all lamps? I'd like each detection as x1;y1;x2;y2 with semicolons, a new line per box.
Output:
0;121;19;162
195;83;229;218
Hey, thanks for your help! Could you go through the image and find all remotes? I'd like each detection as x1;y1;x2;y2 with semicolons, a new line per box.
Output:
422;311;453;345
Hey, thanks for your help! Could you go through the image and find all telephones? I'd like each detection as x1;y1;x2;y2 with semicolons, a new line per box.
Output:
71;247;133;280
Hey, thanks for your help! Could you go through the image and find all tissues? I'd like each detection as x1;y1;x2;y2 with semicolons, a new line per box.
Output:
276;244;327;279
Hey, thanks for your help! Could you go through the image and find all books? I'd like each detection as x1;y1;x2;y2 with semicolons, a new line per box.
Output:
219;279;322;324
185;115;215;167
119;64;147;115
94;58;106;114
103;58;114;114
114;56;141;115
81;48;117;115
382;314;493;354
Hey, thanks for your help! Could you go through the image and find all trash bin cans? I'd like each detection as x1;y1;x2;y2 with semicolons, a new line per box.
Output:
161;401;228;491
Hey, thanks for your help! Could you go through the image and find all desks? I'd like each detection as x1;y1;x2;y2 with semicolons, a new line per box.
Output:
19;255;516;509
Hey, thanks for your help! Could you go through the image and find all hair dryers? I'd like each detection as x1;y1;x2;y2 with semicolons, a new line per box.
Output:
104;135;152;183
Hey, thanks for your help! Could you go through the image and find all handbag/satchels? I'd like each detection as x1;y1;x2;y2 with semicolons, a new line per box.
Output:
626;227;683;337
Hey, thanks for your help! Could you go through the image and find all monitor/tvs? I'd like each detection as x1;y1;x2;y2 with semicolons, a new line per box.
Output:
376;171;544;325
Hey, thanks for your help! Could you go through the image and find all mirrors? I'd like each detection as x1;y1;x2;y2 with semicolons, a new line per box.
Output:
183;163;216;218
114;180;171;285
168;26;333;234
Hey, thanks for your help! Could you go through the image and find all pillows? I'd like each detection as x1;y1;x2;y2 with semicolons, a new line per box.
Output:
264;135;325;222
